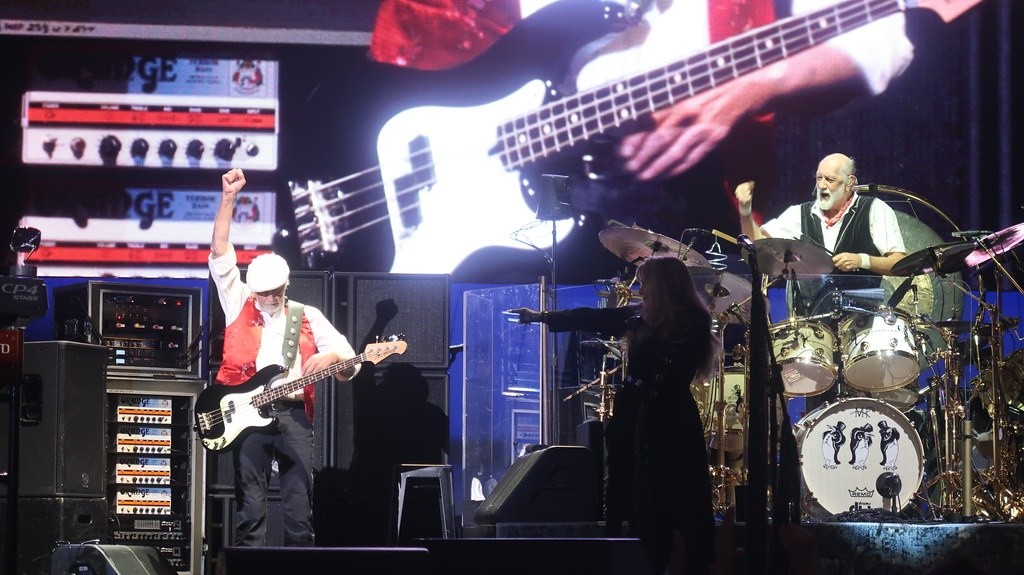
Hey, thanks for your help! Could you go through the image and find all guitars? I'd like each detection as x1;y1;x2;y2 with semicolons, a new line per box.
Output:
193;334;409;454
269;0;980;281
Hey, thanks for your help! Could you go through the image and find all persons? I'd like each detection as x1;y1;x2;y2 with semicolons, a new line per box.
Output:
371;0;915;282
735;154;907;414
208;168;361;548
507;257;724;575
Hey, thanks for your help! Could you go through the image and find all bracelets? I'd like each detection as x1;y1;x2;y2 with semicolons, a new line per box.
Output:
858;253;871;269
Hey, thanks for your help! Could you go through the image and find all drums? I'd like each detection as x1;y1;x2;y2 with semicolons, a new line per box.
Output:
837;306;922;392
768;320;839;398
701;363;788;457
790;397;926;521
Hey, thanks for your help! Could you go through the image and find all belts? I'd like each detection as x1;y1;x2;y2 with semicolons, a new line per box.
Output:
260;401;305;411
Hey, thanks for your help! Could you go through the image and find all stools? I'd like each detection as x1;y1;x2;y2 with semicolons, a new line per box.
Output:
388;463;453;548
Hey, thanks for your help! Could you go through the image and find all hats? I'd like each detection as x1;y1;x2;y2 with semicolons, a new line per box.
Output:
246;254;290;293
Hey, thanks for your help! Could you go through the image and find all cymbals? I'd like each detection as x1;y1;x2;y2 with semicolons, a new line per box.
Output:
890;221;1024;277
598;226;770;324
741;237;835;279
919;317;1021;337
